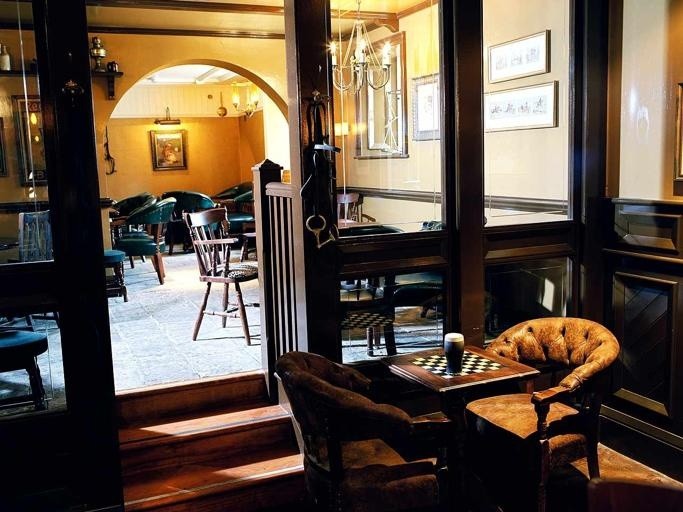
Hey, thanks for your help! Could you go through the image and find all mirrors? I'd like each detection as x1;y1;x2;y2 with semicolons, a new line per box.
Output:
355;33;408;159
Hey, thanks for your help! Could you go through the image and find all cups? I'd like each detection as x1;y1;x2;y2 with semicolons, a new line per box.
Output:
444;333;464;375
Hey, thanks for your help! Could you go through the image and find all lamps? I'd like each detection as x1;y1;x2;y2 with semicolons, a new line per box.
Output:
233;82;260;122
329;0;392;95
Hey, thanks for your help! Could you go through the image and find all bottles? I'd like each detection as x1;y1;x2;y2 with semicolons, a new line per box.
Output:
1;45;11;71
23;214;53;263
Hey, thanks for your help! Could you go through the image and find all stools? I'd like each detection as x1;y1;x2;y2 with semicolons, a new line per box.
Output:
103;250;127;302
0;332;48;413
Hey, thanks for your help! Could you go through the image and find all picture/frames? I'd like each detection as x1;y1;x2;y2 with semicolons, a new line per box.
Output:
12;95;47;187
411;73;441;140
150;129;187;171
488;30;549;84
0;118;8;177
483;80;557;133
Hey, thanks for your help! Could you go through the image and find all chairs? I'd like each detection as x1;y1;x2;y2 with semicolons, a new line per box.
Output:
276;352;453;512
464;317;620;511
365;269;444;346
4;209;60;332
182;204;258;345
210;181;254;250
338;226;400;356
336;193;363;300
162;191;217;255
110;196;177;285
109;194;157;268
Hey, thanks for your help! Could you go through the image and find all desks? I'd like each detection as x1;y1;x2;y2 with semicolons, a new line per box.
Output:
381;344;542;512
